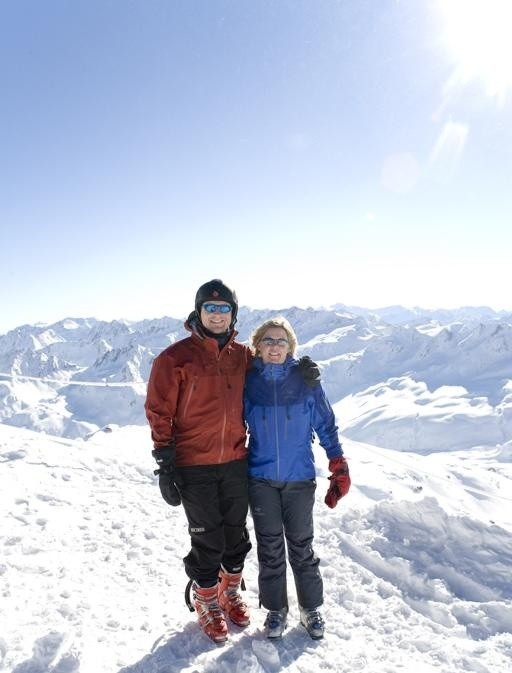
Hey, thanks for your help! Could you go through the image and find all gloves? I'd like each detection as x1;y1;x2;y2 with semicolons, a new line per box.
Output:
297;354;322;387
150;444;183;508
323;454;353;509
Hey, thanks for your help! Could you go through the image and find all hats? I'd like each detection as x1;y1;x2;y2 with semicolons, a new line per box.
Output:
194;277;237;316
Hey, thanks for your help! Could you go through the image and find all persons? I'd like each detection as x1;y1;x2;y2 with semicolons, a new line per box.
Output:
238;315;351;642
144;279;324;645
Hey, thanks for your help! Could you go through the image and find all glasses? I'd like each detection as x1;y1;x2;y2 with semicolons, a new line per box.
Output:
259;336;289;346
201;302;232;314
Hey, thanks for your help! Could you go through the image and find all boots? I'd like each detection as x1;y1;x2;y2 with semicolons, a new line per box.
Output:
218;562;251;624
192;579;228;640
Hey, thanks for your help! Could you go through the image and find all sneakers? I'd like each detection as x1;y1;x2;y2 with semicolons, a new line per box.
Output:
297;602;326;638
262;599;291;638
